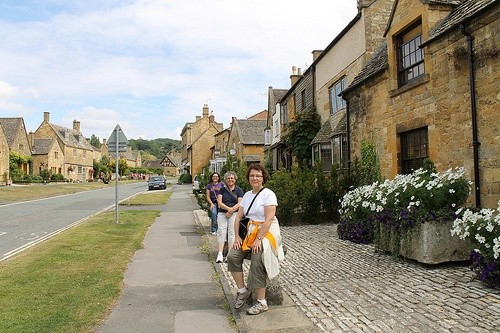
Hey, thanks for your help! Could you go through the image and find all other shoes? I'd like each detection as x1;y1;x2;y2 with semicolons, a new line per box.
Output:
212;232;216;235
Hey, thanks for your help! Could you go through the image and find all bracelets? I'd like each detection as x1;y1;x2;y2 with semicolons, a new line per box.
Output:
257;235;262;240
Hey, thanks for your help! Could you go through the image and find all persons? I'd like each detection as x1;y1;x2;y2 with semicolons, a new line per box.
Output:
207;164;284;316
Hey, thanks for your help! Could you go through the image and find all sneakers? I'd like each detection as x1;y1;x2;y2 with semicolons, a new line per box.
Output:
234;287;252;309
216;252;223;263
246;301;268;314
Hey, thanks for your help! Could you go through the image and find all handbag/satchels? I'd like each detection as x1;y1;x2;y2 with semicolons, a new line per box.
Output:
239;215;250;239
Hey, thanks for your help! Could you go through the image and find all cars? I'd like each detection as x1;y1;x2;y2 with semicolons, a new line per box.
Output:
192;173;205;193
146;176;168;190
